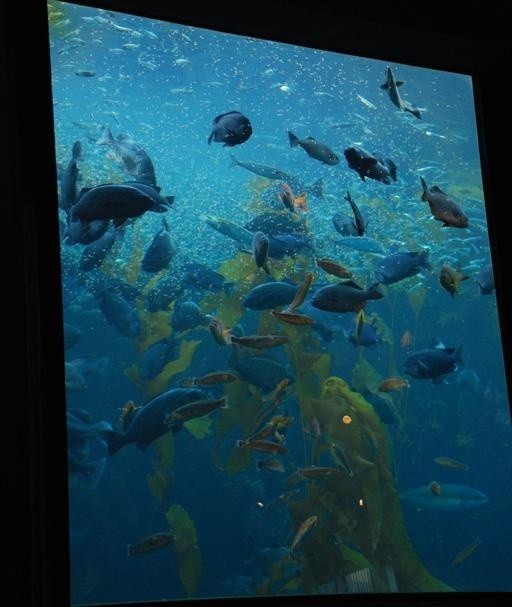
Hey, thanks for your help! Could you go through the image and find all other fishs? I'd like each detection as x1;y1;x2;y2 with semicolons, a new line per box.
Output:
48;12;495;569
